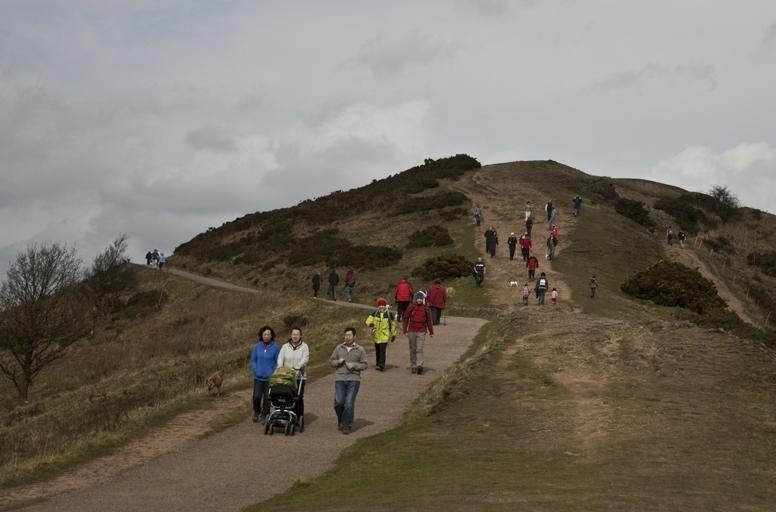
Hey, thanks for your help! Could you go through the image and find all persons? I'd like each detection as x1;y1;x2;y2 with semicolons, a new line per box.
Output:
251;325;280;425
469;198;560;307
395;276;414;320
678;228;688;248
330;327;368;435
328;267;340;301
145;249;165;269
312;269;323;297
345;266;356;303
667;225;675;245
572;193;583;217
426;277;447;327
588;274;599;298
365;296;397;372
401;289;436;375
277;328;311;426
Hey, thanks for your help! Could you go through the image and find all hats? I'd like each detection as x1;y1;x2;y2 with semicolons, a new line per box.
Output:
377;299;387;306
415;291;427;305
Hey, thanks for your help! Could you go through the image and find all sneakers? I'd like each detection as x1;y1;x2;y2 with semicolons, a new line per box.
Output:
260;414;266;424
417;366;423;374
338;423;343;429
375;363;386;370
411;367;416;373
342;426;352;434
252;410;261;422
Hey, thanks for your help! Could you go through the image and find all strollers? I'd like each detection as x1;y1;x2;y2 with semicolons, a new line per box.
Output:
261;364;306;436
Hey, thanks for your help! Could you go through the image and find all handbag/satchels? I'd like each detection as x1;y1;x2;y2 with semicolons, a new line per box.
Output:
347;277;356;288
471;267;477;277
